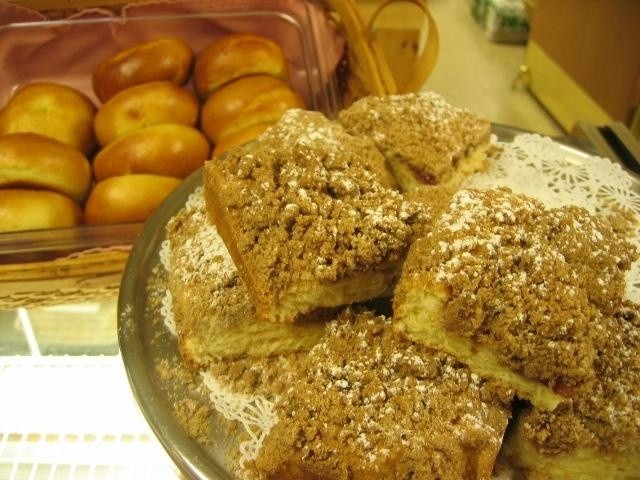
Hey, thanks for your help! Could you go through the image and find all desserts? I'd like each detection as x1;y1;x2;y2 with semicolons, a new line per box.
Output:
160;89;638;480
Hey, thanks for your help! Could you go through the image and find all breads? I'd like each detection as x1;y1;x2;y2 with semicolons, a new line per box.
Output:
0;36;303;242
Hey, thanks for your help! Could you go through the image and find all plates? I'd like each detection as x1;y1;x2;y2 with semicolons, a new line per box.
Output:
116;114;639;480
